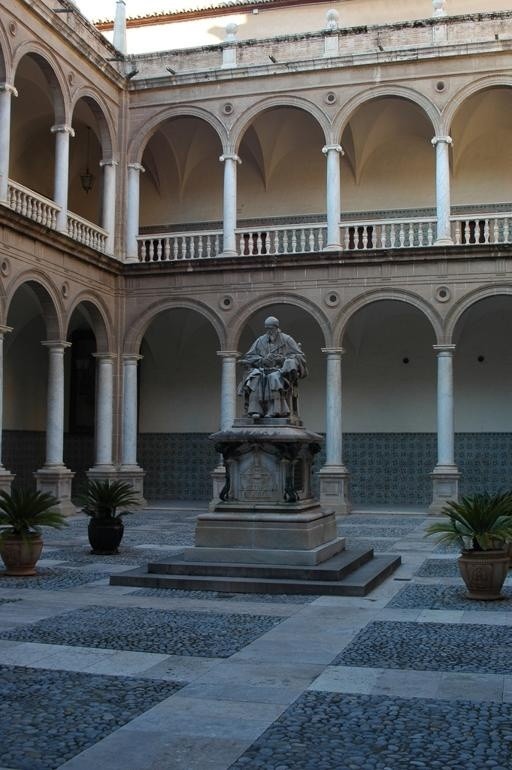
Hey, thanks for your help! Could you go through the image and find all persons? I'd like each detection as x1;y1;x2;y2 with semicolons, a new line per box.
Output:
237;315;309;419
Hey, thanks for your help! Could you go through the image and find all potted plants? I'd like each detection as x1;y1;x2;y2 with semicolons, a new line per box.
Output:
0;484;70;575
423;488;512;600
72;479;142;555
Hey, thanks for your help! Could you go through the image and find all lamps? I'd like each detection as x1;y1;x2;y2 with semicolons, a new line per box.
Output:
78;127;96;196
